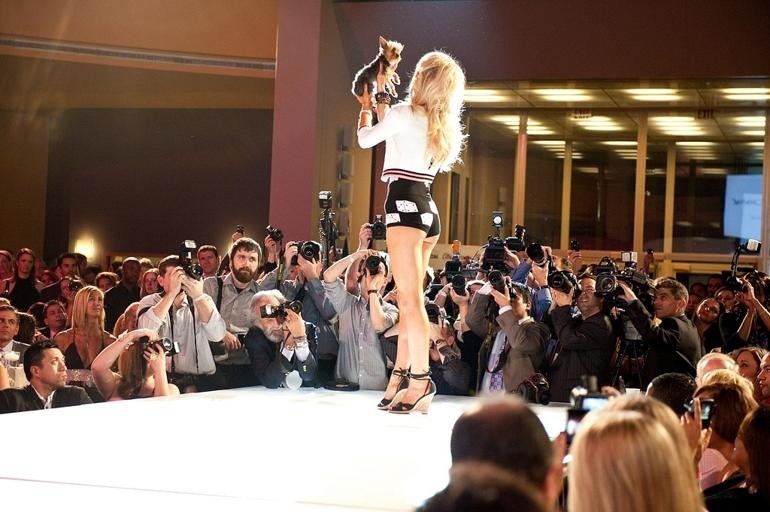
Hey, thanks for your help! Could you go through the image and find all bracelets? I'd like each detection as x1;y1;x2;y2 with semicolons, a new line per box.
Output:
374;92;392;104
359;110;372;117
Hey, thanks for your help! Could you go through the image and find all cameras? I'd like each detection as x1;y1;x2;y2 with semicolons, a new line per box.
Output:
68;280;83;291
368;214;387;240
266;224;284;242
487;270;518;300
236;225;246;234
575;394;617;411
726;275;744;292
526;243;556;275
425;303;440;325
687;398;714;431
139;335;181;356
564;409;591;447
547;270;583;299
451;274;467;297
259;300;303;319
362;256;380;277
288;240;322;264
181;239;204;280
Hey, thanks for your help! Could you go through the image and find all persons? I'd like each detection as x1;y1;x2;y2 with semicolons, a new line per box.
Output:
352;52;471;414
0;223;769;511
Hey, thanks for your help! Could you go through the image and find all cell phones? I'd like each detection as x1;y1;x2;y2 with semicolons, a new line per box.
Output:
569;240;580;252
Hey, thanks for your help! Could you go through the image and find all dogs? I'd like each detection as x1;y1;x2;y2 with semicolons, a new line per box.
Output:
352;36;403;108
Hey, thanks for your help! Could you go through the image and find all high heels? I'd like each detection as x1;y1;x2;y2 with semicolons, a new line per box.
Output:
376;368;436;416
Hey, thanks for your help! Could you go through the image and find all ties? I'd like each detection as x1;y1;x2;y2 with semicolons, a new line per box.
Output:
489;340;507;396
